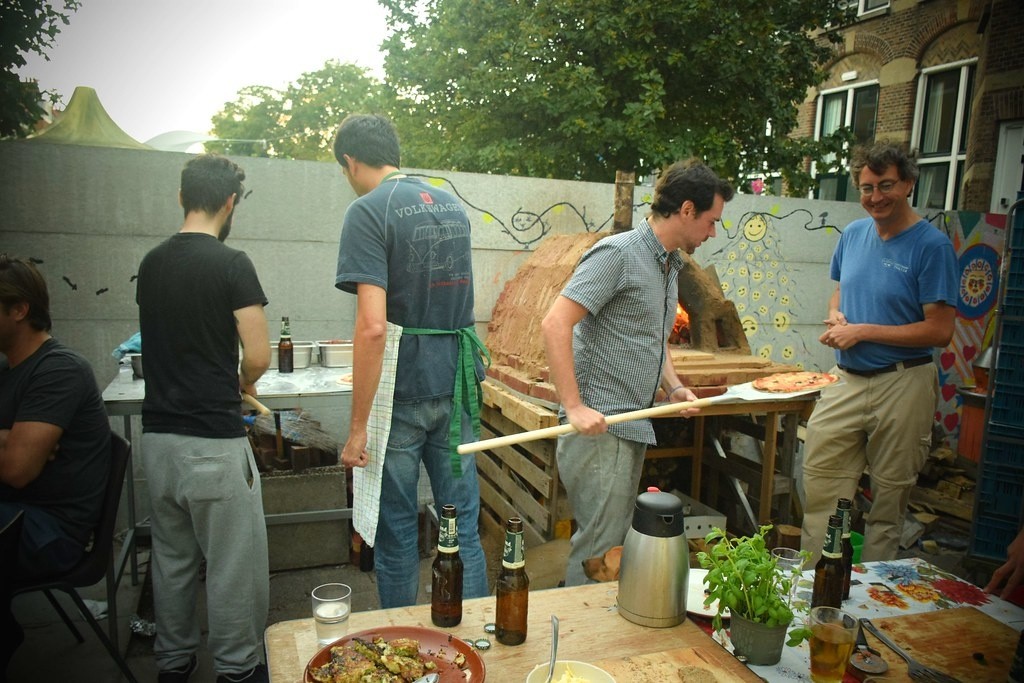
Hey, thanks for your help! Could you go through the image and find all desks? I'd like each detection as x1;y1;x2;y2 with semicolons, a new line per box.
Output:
265;557;1024;683
101;364;376;594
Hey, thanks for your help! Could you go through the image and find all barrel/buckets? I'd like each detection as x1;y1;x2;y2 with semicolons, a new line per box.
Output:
850;530;865;564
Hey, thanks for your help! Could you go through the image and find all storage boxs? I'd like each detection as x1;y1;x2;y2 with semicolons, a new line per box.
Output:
255;456;349;573
965;192;1024;563
668;488;727;539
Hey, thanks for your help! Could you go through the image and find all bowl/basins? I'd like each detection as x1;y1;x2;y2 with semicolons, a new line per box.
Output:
125;352;144;379
526;660;617;683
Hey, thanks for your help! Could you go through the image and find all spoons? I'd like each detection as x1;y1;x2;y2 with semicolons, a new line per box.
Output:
413;672;440;683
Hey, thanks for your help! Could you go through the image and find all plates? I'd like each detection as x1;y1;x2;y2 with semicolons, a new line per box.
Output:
687;567;745;619
303;625;485;683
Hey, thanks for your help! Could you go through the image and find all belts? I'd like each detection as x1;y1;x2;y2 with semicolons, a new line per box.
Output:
837;355;932;377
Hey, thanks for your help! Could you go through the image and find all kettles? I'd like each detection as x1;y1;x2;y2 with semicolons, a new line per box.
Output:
617;487;690;628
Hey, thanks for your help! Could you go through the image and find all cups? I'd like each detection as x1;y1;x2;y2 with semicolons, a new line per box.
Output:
810;606;860;683
311;582;352;650
770;547;803;607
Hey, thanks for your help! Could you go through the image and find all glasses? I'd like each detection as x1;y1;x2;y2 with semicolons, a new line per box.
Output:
860;178;901;196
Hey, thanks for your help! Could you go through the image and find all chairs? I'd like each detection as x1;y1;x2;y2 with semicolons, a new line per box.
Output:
5;429;142;683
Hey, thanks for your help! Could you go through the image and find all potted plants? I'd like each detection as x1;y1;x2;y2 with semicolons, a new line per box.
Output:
684;523;815;666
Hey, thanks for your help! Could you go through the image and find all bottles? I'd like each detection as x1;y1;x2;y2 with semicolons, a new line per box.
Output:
494;516;529;647
1007;629;1024;683
836;497;854;601
360;541;374;573
278;316;294;373
431;504;463;628
811;515;846;610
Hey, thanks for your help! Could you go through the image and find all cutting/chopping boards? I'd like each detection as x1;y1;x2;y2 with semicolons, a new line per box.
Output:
845;606;1022;683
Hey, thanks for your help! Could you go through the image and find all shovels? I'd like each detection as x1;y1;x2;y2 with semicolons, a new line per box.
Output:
456;372;847;455
859;617;963;683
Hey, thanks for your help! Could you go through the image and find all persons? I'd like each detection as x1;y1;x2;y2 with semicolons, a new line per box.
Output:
983;527;1024;600
799;134;959;572
0;254;112;661
136;154;271;683
332;112;490;611
542;156;735;589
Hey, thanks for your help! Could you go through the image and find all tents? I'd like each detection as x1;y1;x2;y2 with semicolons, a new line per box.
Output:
25;88;154;149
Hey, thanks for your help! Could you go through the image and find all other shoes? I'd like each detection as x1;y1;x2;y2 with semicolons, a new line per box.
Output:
158;655;199;683
0;621;24;674
217;663;269;683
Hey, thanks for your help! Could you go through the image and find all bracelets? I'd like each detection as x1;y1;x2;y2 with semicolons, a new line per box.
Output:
665;385;684;403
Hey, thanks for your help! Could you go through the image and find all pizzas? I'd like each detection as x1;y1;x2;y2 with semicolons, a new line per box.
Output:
337;373;354;385
308;633;425;683
752;371;840;393
